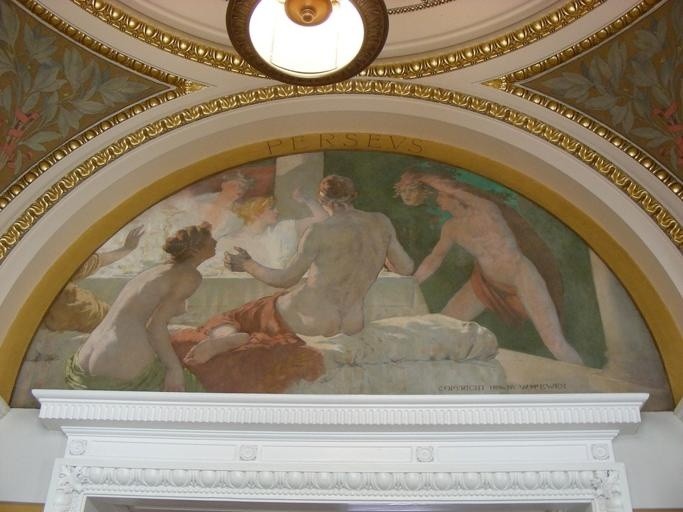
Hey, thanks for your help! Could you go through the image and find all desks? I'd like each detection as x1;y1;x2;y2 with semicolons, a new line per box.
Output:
31;387;648;512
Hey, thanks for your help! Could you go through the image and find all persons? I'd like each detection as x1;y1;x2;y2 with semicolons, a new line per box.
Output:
183;174;415;367
65;221;218;393
174;167;250;241
390;168;462;314
392;168;584;366
212;183;329;270
45;222;146;333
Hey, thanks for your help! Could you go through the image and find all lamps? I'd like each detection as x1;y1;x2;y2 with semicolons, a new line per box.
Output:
226;0;388;85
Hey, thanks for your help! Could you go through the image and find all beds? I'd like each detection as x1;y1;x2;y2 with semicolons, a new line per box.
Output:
9;266;676;411
